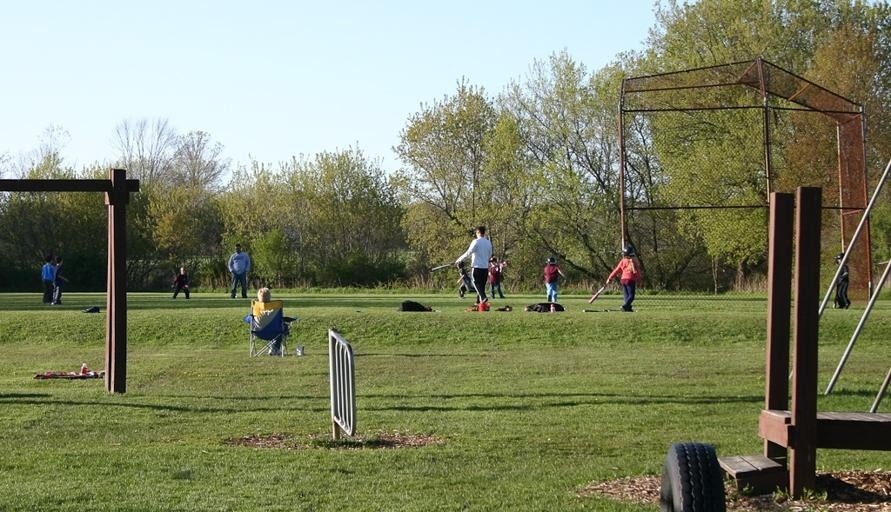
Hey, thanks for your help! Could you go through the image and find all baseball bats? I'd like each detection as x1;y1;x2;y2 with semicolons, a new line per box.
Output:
431;262;457;272
603;309;622;311
588;279;611;304
583;309;607;313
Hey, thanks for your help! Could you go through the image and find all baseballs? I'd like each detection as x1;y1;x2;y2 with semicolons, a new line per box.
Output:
564;308;566;310
507;308;510;311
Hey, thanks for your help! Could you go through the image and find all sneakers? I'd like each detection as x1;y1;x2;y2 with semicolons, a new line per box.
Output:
621;305;633;312
482;298;488;304
269;349;282;355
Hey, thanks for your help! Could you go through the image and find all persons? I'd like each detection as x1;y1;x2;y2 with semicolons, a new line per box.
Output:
544;258;566;303
171;266;192;299
227;244;250;299
50;257;68;305
836;254;851;309
41;256;54;303
457;268;477;297
606;246;642;312
488;256;505;299
249;287;289;355
454;227;492;306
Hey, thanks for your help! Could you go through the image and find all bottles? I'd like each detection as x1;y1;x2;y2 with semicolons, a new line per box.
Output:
81;361;88;375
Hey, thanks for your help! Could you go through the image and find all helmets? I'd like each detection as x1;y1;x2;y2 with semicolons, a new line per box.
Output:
622;246;635;257
488;257;497;263
546;257;557;265
837;253;845;259
